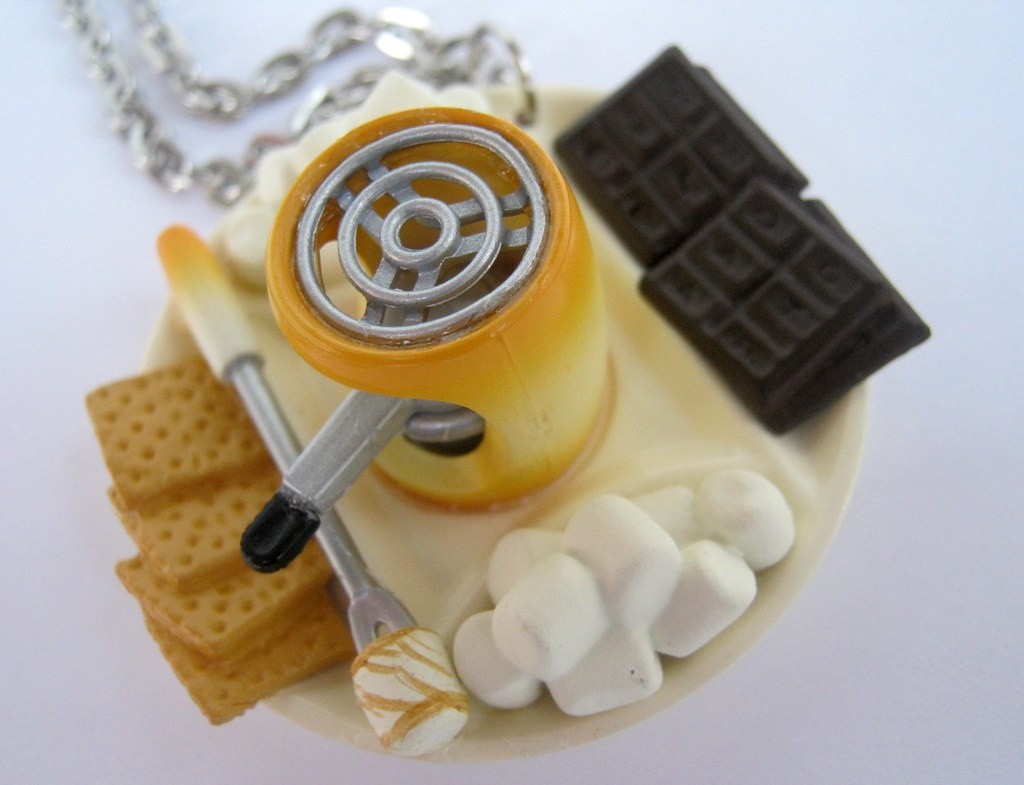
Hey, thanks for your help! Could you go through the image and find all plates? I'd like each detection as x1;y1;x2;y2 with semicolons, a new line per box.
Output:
149;87;867;764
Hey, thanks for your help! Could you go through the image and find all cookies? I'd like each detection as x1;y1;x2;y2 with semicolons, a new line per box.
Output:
552;45;932;432
86;356;352;725
